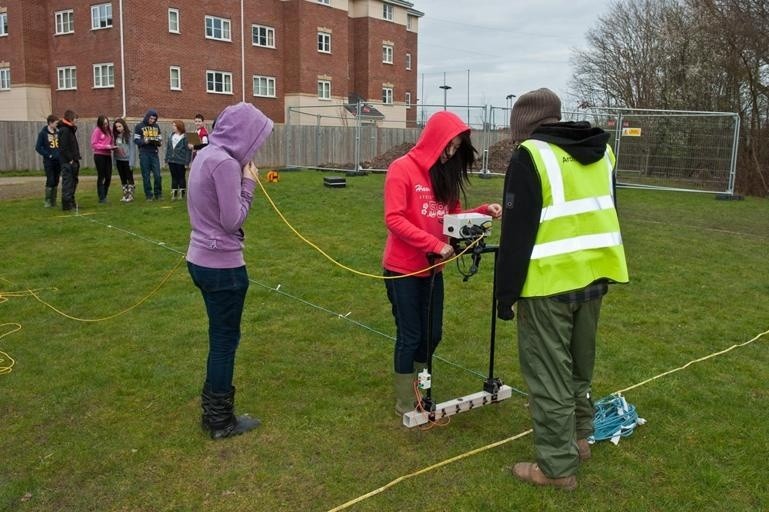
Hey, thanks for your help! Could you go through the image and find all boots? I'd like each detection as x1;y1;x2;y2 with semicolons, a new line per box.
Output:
51;186;58;206
170;188;186;202
127;184;136;202
120;184;128;203
415;359;439;413
43;186;52;208
196;383;262;439
390;368;431;426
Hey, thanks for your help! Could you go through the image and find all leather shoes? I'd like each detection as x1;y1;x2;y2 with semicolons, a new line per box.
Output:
511;460;580;490
578;438;592;460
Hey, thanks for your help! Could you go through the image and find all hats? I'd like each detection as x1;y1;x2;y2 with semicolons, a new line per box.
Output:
509;88;563;146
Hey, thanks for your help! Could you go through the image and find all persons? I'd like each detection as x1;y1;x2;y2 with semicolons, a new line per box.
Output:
34;114;60;208
132;109;164;202
185;100;274;441
494;86;630;492
187;113;209;151
382;110;503;417
56;109;81;211
164;119;192;200
112;118;136;202
90;114;114;203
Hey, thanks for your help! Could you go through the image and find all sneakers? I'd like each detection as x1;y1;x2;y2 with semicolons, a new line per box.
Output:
62;203;78;211
99;198;109;203
146;197;153;202
156;197;165;202
